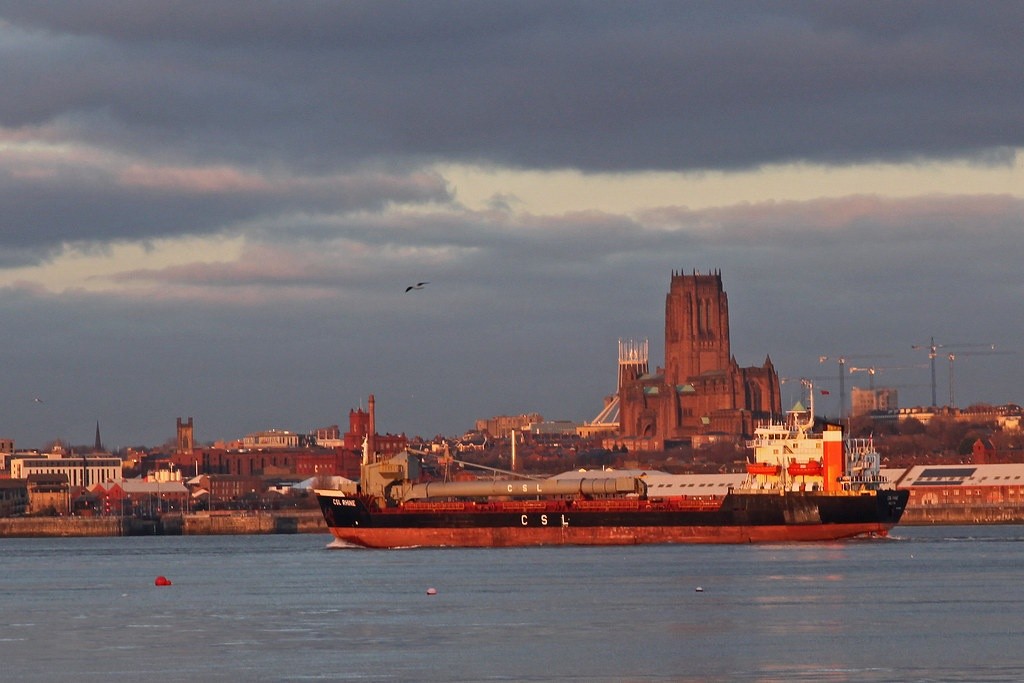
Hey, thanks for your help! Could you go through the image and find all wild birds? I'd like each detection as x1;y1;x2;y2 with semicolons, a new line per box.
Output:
28;398;44;404
405;282;430;293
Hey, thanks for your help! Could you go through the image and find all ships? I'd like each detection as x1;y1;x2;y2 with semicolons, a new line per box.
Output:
314;379;910;551
